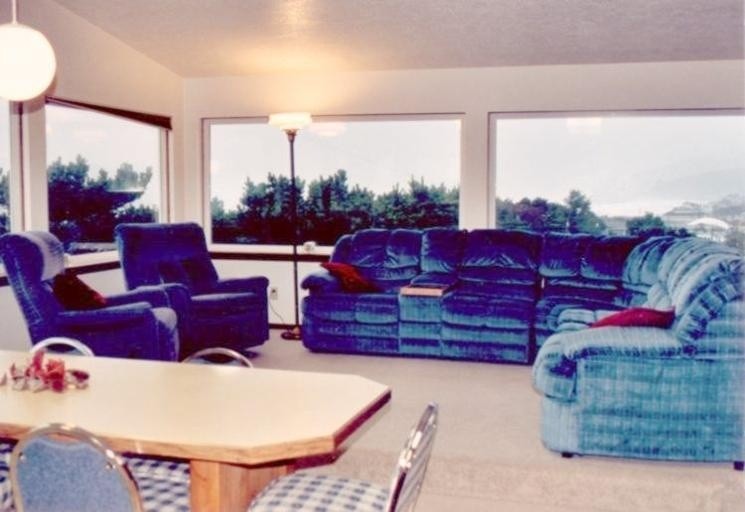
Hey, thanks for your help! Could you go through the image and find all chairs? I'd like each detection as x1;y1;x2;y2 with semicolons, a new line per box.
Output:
239;400;440;512
177;348;254;370
1;228;182;368
6;421;149;512
26;334;97;360
113;219;272;356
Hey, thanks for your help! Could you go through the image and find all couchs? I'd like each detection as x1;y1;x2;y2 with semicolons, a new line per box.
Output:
301;225;538;366
539;224;737;471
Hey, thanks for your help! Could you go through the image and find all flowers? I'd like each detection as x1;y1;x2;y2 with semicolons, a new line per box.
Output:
0;348;92;393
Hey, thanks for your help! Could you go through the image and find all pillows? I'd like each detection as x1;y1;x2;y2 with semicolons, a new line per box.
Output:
51;271;109;314
589;303;676;329
319;257;381;294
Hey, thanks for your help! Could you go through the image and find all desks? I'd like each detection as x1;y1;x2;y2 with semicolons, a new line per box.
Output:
0;346;393;512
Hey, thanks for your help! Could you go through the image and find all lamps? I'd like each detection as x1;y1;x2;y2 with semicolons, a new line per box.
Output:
268;114;311;341
1;0;57;102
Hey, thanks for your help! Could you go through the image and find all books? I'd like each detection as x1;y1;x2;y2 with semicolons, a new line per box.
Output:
400;282;451;297
